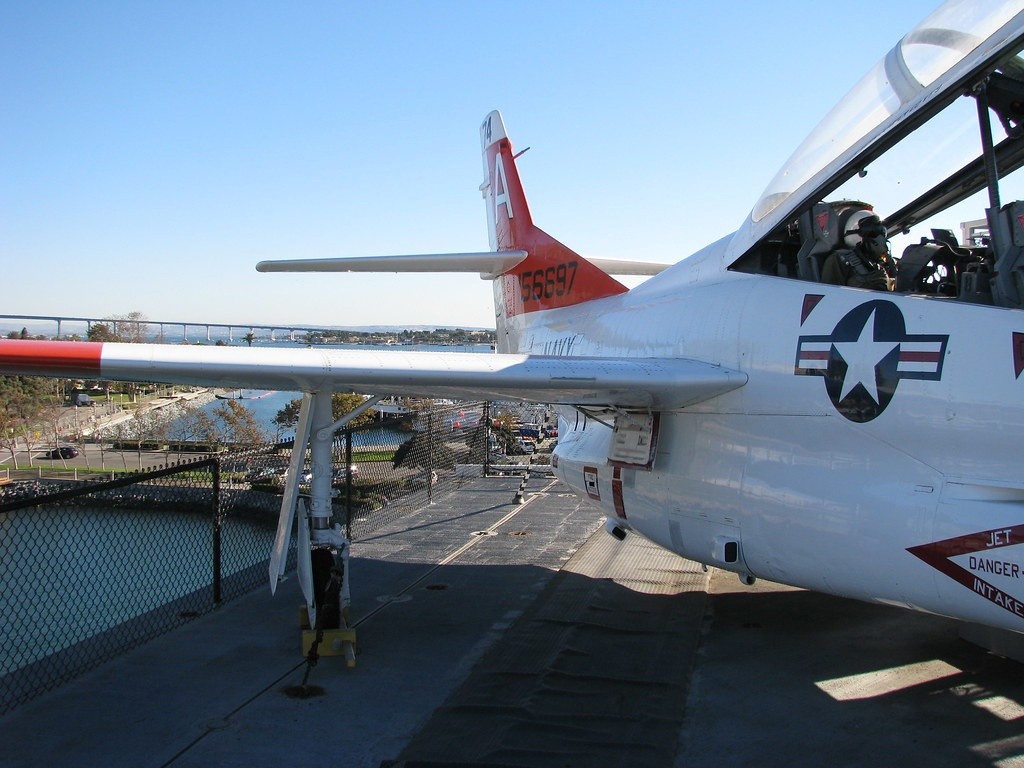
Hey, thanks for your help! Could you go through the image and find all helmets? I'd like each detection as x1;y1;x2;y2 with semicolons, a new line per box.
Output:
844;209;887;249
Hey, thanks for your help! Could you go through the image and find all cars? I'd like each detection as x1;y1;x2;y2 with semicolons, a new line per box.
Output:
226;466;359;484
45;446;78;460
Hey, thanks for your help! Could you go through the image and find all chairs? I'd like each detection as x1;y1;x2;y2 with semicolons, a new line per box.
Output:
822;208;897;288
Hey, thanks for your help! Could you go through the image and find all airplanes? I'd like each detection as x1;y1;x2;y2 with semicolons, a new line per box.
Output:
0;0;1024;663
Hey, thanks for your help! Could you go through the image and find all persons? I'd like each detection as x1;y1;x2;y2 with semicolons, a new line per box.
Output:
821;210;899;292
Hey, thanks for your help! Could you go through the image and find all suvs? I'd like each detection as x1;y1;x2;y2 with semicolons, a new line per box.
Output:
73;393;97;407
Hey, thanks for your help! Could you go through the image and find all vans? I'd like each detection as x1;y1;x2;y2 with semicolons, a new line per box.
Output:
514;440;535;454
